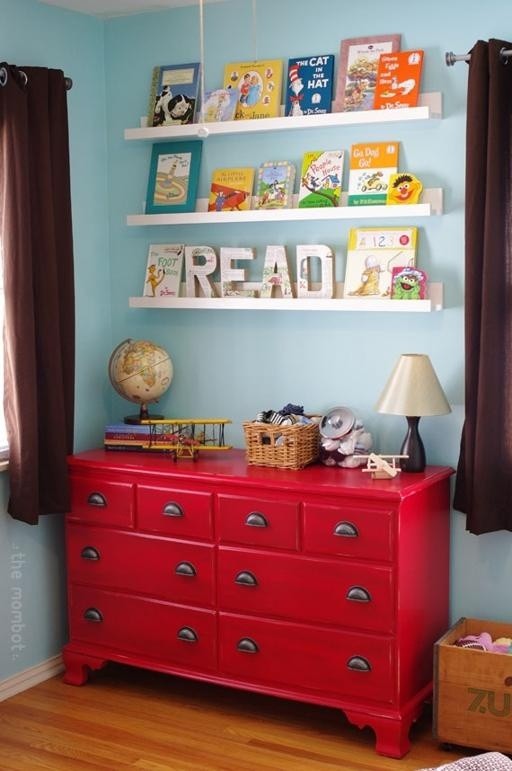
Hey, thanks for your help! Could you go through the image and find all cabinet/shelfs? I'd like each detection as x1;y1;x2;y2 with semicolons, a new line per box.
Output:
122;91;445;314
61;449;457;760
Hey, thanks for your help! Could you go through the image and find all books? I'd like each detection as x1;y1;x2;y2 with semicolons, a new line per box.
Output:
345;140;400;207
200;88;238;122
373;50;423;109
254;161;296;210
207;168;255;211
224;59;283;119
103;422;188;454
333;33;400;113
298;149;344;208
285;55;334;116
144;141;205;214
342;227;417;297
145;63;200;126
387;265;427;300
142;243;185;297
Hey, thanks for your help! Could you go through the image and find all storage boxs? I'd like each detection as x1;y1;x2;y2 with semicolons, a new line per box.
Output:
430;616;512;759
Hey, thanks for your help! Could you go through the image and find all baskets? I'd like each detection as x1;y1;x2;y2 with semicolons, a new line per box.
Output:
243;410;326;471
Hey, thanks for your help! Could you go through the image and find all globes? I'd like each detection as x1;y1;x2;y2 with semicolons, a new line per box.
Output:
108;338;175;426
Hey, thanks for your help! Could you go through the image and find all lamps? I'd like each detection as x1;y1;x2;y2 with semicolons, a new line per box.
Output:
375;354;453;473
